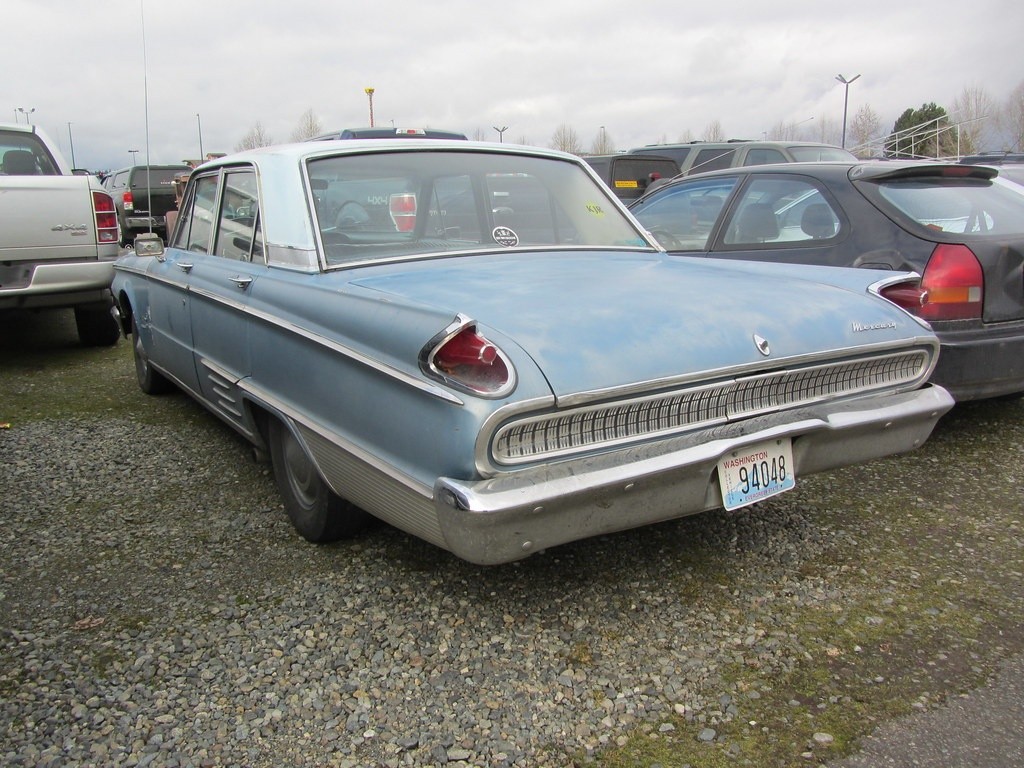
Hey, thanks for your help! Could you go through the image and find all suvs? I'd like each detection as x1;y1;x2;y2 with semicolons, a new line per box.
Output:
101;165;197;249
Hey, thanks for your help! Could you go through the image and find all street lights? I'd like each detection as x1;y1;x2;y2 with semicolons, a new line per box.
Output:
128;150;139;166
495;126;509;143
835;73;861;149
17;106;36;123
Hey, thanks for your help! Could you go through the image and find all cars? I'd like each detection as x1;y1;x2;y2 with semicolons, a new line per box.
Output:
625;160;1024;426
301;127;1024;243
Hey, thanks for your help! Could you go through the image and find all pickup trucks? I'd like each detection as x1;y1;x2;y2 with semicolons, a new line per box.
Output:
180;181;266;266
0;123;121;350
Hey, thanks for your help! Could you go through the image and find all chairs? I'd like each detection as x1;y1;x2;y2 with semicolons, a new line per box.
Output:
4;150;34;175
740;202;780;242
802;203;835;240
